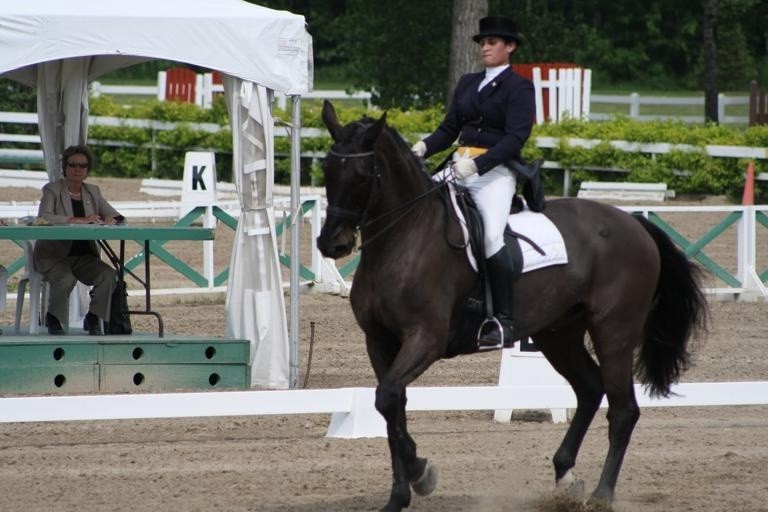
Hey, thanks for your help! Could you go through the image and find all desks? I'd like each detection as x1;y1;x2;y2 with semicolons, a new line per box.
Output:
2;226;217;339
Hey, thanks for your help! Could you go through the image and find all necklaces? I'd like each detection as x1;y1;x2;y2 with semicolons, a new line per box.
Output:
69;191;81;197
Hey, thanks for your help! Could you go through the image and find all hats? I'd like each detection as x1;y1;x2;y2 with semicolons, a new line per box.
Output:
472;15;523;45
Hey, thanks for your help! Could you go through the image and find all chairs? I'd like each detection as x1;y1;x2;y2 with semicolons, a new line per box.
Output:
13;214;107;336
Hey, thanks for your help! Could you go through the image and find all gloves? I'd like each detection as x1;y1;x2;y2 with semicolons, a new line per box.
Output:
453;158;478;180
411;140;426;158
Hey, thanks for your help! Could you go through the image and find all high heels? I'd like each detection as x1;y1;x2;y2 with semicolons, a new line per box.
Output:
83;311;104;335
44;312;66;334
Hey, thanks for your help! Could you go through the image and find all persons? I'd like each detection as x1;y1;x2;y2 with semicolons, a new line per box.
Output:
32;144;128;335
411;15;537;348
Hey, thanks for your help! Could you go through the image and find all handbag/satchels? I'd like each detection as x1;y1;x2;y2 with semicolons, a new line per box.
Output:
89;279;132;335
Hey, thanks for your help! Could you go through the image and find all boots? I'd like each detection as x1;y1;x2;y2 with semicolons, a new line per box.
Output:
473;243;514;348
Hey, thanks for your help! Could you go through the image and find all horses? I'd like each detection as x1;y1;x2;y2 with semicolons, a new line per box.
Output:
316;98;714;512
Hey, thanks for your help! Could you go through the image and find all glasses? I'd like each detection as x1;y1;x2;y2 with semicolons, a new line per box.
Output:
64;159;89;169
